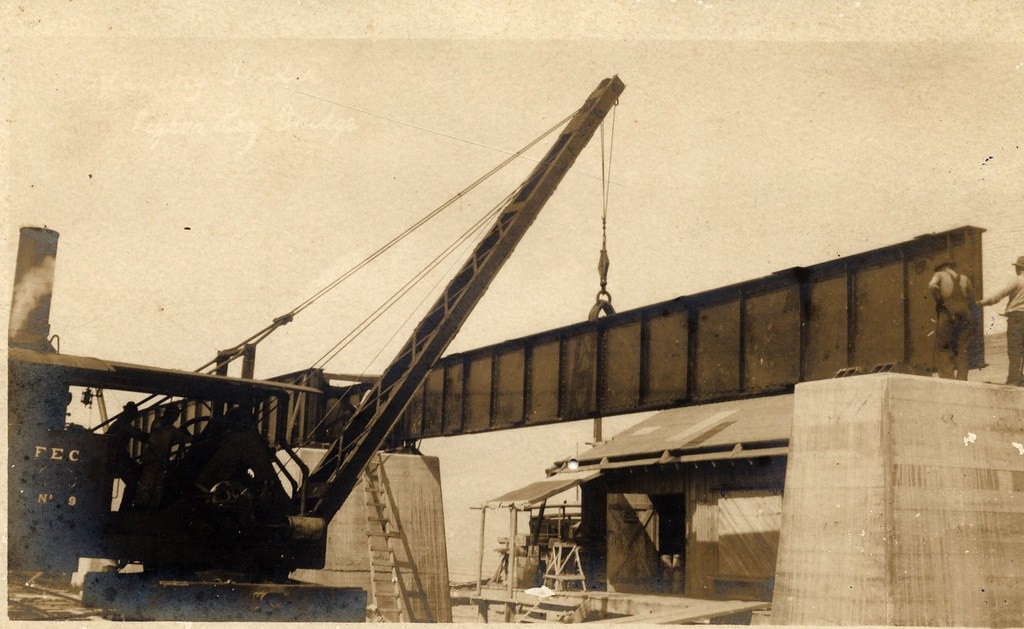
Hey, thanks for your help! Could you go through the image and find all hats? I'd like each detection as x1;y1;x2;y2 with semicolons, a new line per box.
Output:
1012;256;1024;268
933;259;956;272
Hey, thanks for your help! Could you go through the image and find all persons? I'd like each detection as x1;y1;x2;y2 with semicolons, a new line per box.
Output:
103;400;152;508
926;251;978;383
131;400;190;509
976;254;1024;388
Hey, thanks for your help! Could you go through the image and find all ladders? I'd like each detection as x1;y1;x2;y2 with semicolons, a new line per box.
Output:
354;453;405;624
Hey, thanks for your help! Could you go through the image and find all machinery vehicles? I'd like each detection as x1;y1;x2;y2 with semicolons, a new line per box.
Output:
22;73;629;621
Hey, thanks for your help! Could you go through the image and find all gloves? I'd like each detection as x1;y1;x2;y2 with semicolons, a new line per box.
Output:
935;297;944;312
